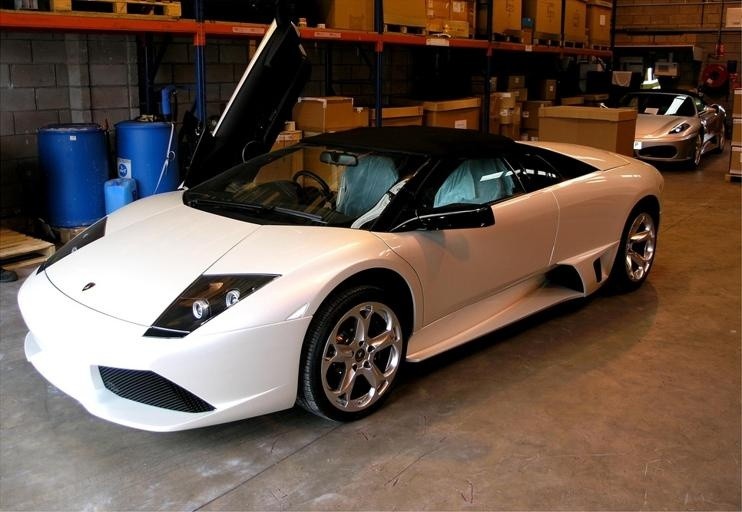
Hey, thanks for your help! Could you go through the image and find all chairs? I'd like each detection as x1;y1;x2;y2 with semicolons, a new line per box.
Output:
338;154;402;219
431;159;515;209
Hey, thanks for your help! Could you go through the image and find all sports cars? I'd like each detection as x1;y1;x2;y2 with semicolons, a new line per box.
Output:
599;86;730;173
12;13;670;437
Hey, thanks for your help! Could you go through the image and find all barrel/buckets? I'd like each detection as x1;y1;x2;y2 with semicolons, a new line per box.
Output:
117;120;175;194
39;125;106;227
102;177;136;213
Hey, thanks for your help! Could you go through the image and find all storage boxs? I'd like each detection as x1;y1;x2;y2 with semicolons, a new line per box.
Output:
323;0;606;48
253;72;640;191
614;0;742;62
729;88;742;175
